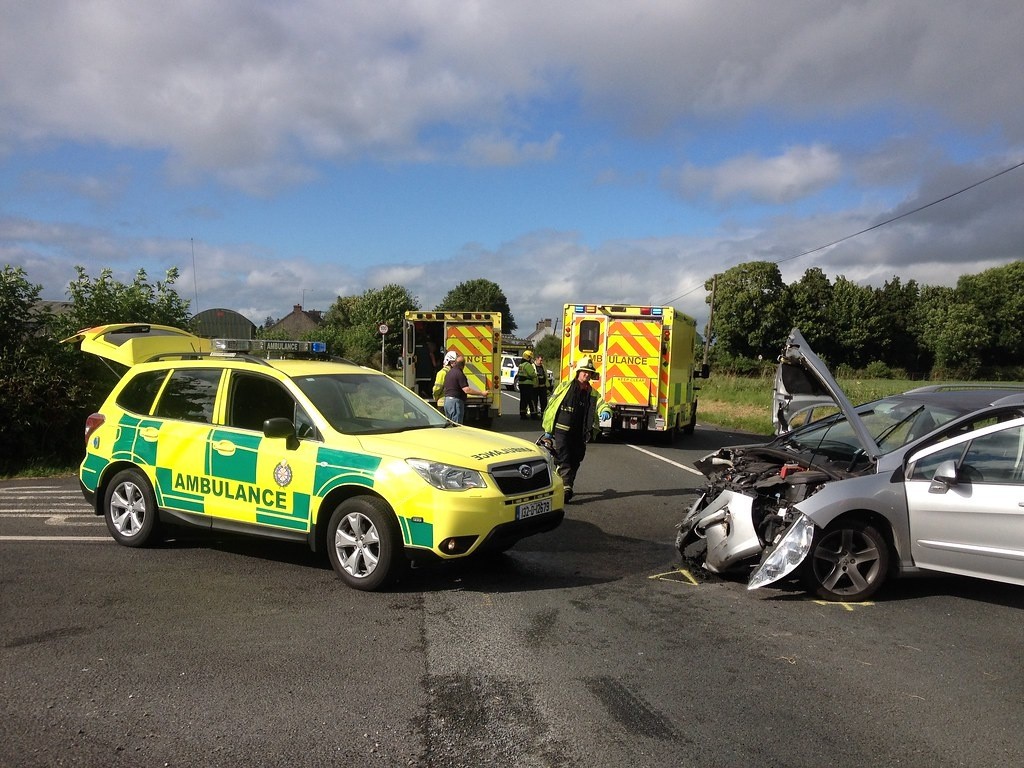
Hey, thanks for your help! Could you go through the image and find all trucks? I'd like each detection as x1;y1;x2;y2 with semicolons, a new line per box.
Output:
401;311;501;428
560;303;709;443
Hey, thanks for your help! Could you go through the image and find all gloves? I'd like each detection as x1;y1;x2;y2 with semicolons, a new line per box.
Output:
545;433;553;439
599;411;610;422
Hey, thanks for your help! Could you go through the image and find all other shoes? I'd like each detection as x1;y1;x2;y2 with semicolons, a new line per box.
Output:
563;487;573;504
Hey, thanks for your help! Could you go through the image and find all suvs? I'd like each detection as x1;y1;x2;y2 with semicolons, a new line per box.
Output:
59;322;564;590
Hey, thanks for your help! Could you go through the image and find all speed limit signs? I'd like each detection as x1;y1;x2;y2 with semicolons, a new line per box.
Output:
377;324;390;335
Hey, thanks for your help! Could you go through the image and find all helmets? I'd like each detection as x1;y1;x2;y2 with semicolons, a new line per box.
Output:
575;358;596;372
443;351;458;367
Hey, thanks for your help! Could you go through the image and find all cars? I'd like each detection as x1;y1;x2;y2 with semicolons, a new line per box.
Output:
674;328;1024;603
499;351;554;394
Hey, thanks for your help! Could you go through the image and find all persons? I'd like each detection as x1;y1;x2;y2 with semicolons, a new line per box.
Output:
542;356;612;501
414;321;444;399
444;356;491;425
518;350;541;420
432;351;460;416
531;354;547;416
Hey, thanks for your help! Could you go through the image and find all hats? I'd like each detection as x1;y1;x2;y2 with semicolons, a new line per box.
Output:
522;350;534;361
